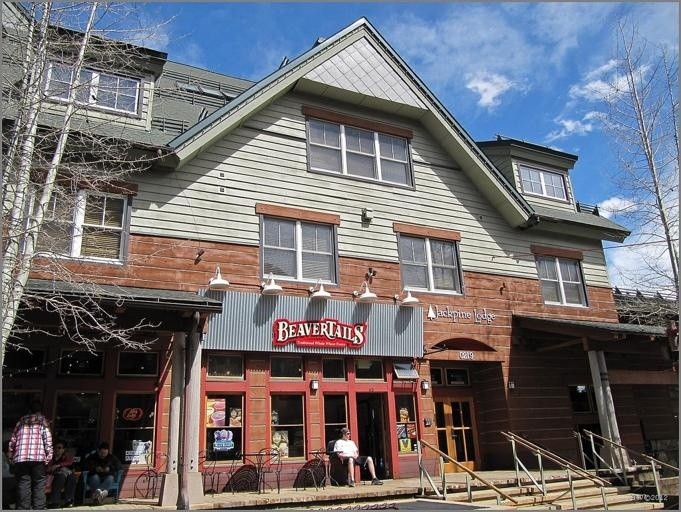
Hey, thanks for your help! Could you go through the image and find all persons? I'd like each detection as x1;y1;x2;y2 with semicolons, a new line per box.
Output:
271;432;287;456
229;409;241;426
2;402;119;511
334;427;383;487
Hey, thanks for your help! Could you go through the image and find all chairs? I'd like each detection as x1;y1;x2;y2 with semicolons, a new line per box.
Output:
135;439;367;499
84;450;124;503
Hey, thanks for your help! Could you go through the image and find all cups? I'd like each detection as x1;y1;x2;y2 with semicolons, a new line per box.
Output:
207;398;226;428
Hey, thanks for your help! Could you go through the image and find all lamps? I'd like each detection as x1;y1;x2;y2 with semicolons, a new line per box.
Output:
509;380;515;388
422;380;429;390
209;265;420;305
312;379;319;389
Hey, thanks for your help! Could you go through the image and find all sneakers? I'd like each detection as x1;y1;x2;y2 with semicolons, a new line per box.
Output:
91;489;101;500
371;478;383;485
349;480;355;487
97;490;107;501
